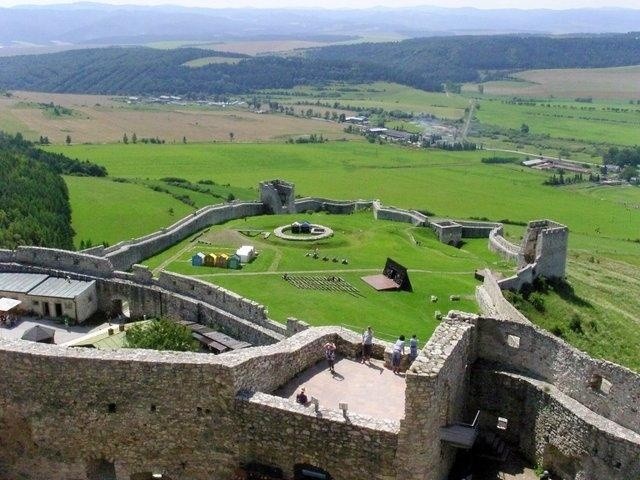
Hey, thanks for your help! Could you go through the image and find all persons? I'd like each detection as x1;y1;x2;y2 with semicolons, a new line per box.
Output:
296;386;311;405
0;311;18;329
392;334;405;374
358;326;374;366
104;302;123;326
409;334;417;363
324;340;336;371
